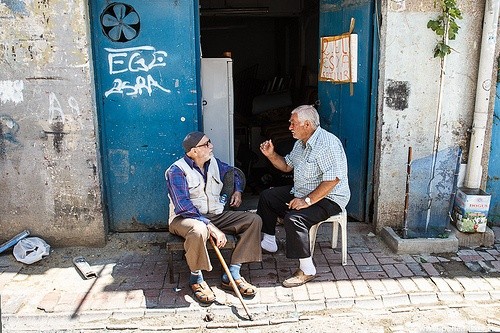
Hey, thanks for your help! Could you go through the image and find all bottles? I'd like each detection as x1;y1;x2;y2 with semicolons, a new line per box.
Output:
219;193;228;204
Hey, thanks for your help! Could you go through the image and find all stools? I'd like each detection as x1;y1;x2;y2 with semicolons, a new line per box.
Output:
309;209;348;265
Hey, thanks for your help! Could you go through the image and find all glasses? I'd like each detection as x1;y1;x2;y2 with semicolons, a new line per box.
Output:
194;139;212;148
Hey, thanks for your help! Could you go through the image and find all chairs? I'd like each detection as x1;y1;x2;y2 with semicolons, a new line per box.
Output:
166;172;236;284
234;63;318;185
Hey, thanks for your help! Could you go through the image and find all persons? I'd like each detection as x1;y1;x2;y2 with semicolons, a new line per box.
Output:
256;105;351;288
165;130;263;304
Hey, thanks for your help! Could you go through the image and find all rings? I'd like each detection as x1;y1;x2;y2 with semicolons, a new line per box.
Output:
219;240;222;243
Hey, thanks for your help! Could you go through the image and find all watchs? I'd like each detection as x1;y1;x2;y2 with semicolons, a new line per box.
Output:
303;195;311;206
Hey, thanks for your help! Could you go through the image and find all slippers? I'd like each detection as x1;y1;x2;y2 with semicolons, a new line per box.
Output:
221;276;258;295
191;281;216;303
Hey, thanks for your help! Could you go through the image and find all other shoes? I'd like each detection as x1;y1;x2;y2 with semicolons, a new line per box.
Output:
283;269;316;287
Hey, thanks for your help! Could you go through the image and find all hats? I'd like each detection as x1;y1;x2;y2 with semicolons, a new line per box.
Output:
183;131;205;153
